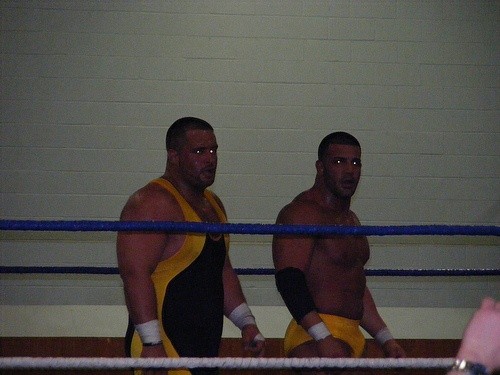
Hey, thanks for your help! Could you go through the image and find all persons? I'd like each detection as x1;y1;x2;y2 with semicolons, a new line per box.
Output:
117;116;266;375
272;131;405;375
446;298;500;375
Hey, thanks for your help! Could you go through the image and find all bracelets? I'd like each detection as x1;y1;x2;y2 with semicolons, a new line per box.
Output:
229;303;256;330
136;320;162;347
308;322;330;342
375;327;393;345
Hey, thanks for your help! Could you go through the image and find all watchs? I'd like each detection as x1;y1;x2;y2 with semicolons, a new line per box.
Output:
448;361;488;375
